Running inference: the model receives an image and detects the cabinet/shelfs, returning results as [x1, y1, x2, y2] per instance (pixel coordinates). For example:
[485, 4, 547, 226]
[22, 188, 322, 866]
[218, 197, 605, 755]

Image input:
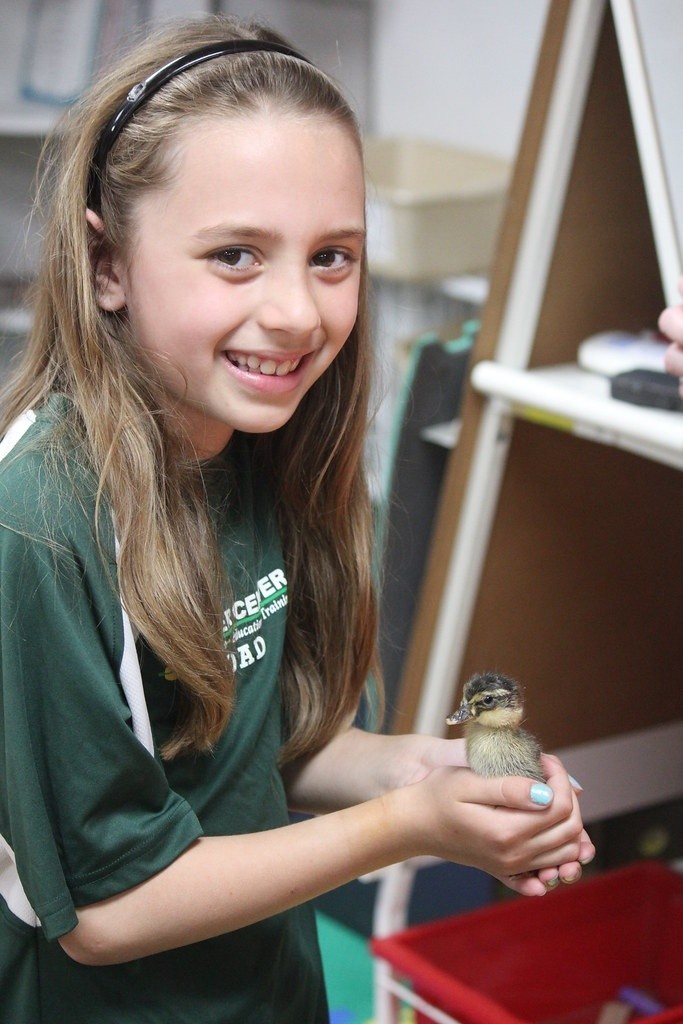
[0, 1, 375, 400]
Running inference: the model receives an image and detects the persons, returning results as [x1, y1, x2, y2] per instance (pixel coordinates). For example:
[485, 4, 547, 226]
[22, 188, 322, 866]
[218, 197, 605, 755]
[0, 14, 594, 1024]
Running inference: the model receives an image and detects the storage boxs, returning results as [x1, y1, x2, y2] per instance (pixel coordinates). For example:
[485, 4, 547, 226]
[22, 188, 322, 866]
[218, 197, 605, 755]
[370, 861, 683, 1024]
[351, 135, 516, 281]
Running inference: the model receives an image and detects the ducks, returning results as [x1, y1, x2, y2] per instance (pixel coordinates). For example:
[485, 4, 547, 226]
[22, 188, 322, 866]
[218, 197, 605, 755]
[444, 672, 547, 783]
